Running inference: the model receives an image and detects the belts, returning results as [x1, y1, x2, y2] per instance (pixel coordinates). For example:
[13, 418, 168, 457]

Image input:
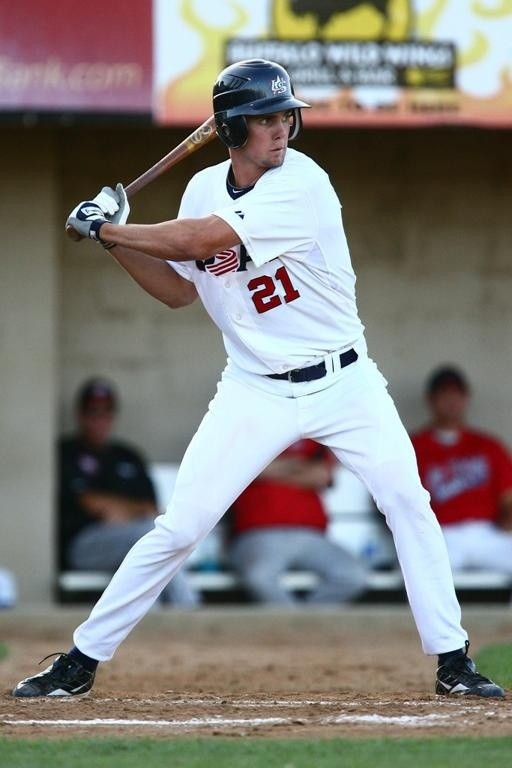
[264, 349, 358, 383]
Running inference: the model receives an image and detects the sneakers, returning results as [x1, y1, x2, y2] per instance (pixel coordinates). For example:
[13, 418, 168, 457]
[11, 653, 97, 700]
[435, 639, 504, 698]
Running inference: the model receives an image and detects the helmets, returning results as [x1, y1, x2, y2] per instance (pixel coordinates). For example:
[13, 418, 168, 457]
[212, 59, 314, 150]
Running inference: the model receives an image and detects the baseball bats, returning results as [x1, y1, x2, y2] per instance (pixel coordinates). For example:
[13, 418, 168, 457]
[66, 114, 218, 242]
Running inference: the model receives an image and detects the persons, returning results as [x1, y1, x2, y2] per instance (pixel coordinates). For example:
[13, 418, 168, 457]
[373, 356, 509, 606]
[41, 372, 202, 612]
[220, 436, 370, 605]
[1, 56, 510, 703]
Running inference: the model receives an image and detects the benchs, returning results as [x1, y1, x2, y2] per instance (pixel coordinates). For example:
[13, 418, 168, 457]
[58, 462, 512, 592]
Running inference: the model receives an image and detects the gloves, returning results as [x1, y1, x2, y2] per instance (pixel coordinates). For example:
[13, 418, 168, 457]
[64, 183, 131, 250]
